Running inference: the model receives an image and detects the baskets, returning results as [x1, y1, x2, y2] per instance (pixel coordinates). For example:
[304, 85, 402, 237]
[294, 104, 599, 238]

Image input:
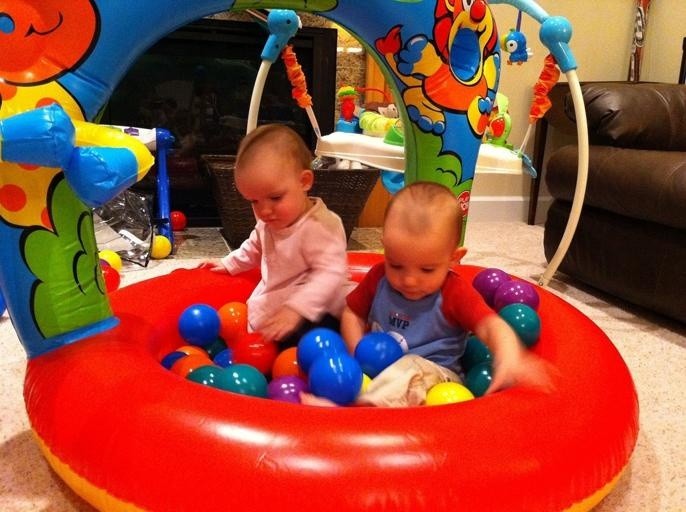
[200, 152, 382, 253]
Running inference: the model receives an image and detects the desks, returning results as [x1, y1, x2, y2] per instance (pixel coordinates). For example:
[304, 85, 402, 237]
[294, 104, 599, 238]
[100, 18, 338, 226]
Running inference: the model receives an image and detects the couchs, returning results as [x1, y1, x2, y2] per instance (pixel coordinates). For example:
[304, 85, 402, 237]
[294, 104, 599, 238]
[544, 83, 686, 326]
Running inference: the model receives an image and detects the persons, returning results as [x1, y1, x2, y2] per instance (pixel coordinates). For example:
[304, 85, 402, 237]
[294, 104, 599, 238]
[300, 181, 565, 409]
[196, 123, 360, 352]
